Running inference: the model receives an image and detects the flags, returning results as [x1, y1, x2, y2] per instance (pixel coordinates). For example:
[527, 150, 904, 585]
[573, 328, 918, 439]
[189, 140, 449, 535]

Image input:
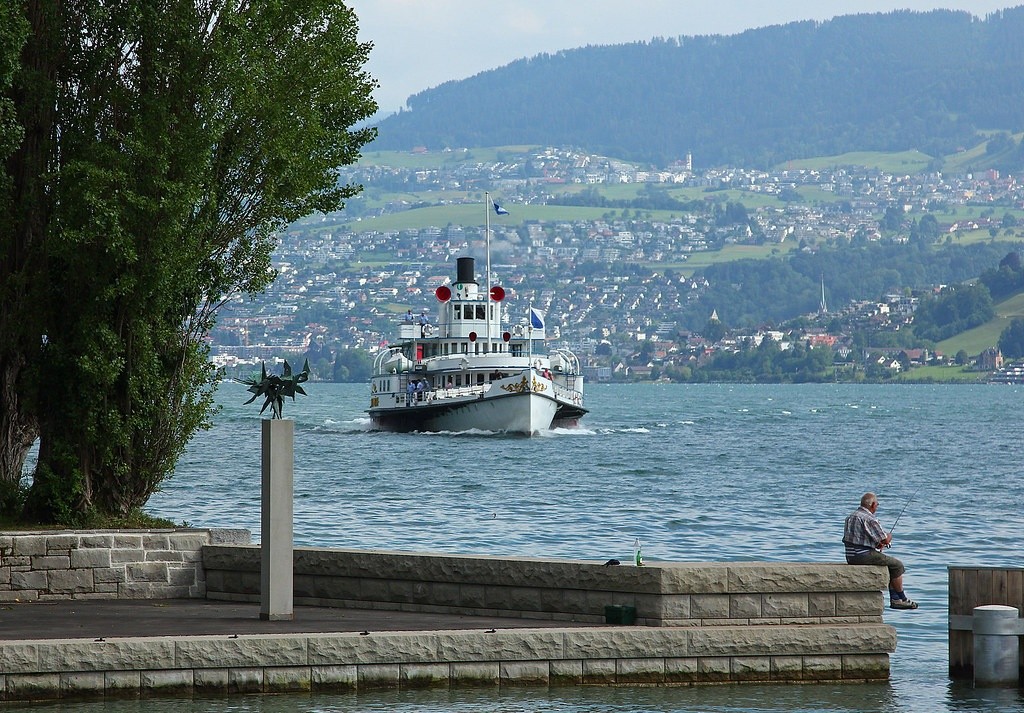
[489, 194, 510, 216]
[531, 306, 547, 329]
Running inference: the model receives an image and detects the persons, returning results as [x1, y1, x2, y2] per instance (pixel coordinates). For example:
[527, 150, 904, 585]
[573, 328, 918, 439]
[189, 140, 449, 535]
[542, 368, 553, 381]
[494, 369, 502, 379]
[446, 379, 453, 389]
[842, 492, 918, 610]
[419, 311, 430, 328]
[404, 309, 415, 325]
[407, 377, 432, 399]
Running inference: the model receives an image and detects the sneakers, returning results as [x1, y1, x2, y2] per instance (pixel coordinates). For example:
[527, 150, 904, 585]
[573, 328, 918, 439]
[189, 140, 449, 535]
[890, 597, 918, 609]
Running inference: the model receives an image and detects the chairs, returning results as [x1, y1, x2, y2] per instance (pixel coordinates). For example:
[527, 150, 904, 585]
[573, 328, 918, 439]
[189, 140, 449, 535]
[427, 383, 491, 400]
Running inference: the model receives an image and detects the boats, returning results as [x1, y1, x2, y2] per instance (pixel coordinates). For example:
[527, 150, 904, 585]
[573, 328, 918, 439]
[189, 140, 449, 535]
[383, 351, 413, 373]
[362, 191, 588, 435]
[550, 353, 574, 375]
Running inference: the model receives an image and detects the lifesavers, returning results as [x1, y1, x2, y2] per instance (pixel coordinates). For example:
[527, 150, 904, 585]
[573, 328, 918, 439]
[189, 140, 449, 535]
[512, 324, 524, 337]
[422, 323, 434, 336]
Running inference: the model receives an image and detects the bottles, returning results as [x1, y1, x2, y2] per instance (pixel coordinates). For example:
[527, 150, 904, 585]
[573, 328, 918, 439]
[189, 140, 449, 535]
[633, 537, 641, 565]
[636, 551, 642, 566]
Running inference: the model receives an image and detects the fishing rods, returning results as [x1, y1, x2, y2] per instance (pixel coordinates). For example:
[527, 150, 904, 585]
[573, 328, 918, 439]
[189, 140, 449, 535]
[883, 482, 927, 549]
